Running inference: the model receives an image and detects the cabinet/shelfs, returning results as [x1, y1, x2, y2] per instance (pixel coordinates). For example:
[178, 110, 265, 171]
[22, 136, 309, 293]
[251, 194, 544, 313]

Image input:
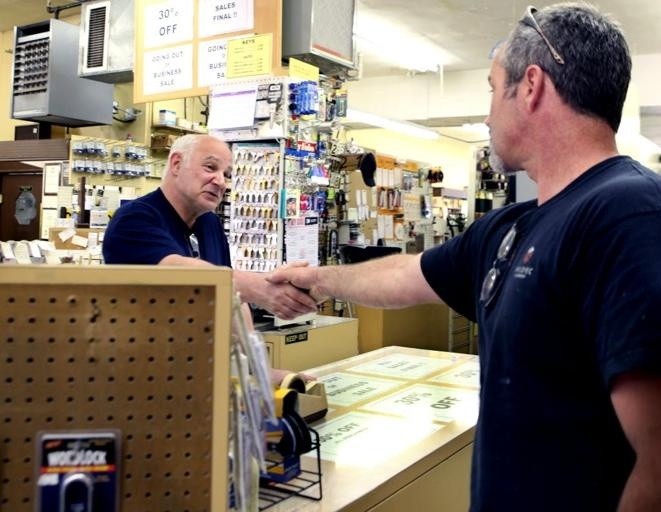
[342, 302, 470, 354]
[251, 316, 359, 375]
[469, 128, 660, 353]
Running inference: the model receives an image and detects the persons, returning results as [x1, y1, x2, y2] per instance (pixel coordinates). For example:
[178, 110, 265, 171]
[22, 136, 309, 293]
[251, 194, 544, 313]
[262, 1, 661, 511]
[100, 133, 321, 389]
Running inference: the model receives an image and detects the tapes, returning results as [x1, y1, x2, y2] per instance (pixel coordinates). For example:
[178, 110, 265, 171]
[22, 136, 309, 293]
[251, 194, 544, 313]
[279, 373, 306, 394]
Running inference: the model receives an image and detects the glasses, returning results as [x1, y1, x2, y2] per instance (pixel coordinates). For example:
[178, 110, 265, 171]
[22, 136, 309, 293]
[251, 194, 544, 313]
[517, 5, 566, 65]
[478, 211, 530, 311]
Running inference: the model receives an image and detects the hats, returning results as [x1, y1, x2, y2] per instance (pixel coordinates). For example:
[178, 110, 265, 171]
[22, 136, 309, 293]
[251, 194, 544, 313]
[358, 152, 378, 188]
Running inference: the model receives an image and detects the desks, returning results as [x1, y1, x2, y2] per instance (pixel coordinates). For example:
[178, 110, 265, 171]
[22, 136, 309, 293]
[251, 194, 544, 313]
[229, 344, 483, 512]
